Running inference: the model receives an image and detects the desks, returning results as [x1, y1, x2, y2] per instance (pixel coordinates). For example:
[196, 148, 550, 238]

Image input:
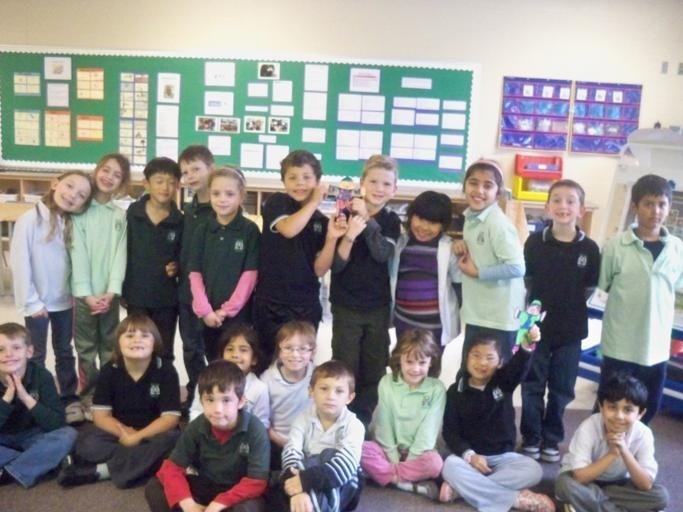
[0, 172, 598, 243]
[0, 203, 43, 297]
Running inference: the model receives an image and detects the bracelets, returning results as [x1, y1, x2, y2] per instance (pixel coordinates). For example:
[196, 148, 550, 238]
[344, 235, 354, 243]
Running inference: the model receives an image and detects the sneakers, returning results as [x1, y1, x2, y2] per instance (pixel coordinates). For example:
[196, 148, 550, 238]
[438, 481, 461, 503]
[542, 448, 560, 463]
[56, 465, 100, 488]
[412, 480, 439, 501]
[518, 489, 556, 512]
[65, 396, 92, 427]
[523, 446, 541, 460]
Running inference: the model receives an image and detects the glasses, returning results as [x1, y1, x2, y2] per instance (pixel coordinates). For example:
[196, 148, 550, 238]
[278, 346, 314, 353]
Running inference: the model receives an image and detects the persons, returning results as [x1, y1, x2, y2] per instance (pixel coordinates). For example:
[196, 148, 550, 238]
[328, 154, 401, 426]
[11, 169, 95, 423]
[0, 311, 668, 510]
[452, 160, 526, 383]
[519, 179, 602, 461]
[186, 166, 262, 364]
[65, 153, 129, 422]
[387, 190, 463, 379]
[120, 157, 184, 363]
[252, 150, 349, 378]
[177, 145, 217, 410]
[592, 175, 682, 425]
